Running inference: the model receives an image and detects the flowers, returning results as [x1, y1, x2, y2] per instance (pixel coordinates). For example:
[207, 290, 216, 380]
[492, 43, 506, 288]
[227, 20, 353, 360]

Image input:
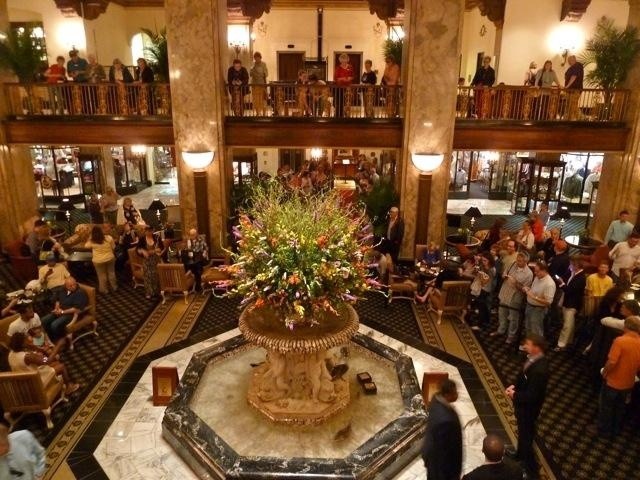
[208, 172, 394, 332]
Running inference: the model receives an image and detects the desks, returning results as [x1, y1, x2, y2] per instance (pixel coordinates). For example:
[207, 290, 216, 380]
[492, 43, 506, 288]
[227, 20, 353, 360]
[565, 235, 603, 255]
[33, 223, 66, 242]
[445, 234, 481, 261]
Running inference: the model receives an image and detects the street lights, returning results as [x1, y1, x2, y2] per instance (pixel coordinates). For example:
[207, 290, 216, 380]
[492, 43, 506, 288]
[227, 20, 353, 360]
[180, 150, 218, 258]
[411, 152, 446, 253]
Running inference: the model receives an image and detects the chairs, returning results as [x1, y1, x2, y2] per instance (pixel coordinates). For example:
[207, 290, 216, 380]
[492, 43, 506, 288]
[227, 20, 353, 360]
[387, 244, 472, 326]
[2, 229, 231, 305]
[1, 280, 99, 428]
[579, 245, 610, 269]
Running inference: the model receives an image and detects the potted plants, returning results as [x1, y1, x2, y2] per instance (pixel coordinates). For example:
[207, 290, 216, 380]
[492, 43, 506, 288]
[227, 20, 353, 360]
[580, 16, 640, 121]
[0, 23, 49, 115]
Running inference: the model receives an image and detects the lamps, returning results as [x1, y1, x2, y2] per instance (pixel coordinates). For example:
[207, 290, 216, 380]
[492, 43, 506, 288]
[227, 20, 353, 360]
[554, 209, 571, 239]
[411, 153, 444, 173]
[58, 199, 76, 235]
[181, 151, 214, 170]
[146, 198, 166, 225]
[464, 207, 483, 236]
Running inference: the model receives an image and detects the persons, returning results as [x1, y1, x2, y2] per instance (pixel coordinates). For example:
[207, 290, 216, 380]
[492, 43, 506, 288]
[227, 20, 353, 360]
[464, 199, 639, 358]
[348, 156, 475, 306]
[8, 333, 80, 395]
[504, 334, 549, 463]
[462, 434, 523, 480]
[332, 54, 355, 118]
[383, 55, 400, 120]
[26, 187, 209, 296]
[1, 252, 91, 367]
[43, 56, 66, 117]
[531, 60, 560, 123]
[563, 55, 583, 121]
[227, 59, 250, 117]
[420, 379, 463, 480]
[523, 61, 537, 86]
[457, 77, 465, 112]
[66, 51, 95, 114]
[132, 57, 154, 115]
[86, 51, 106, 116]
[227, 156, 331, 262]
[468, 55, 496, 116]
[249, 51, 268, 118]
[362, 60, 377, 117]
[0, 415, 45, 480]
[600, 315, 639, 435]
[108, 58, 135, 115]
[296, 72, 326, 119]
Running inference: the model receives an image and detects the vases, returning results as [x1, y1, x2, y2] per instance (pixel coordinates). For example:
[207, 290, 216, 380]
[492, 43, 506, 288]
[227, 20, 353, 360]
[238, 302, 359, 425]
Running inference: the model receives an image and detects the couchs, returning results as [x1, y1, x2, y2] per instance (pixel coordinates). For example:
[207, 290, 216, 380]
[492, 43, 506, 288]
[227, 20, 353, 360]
[64, 223, 147, 252]
